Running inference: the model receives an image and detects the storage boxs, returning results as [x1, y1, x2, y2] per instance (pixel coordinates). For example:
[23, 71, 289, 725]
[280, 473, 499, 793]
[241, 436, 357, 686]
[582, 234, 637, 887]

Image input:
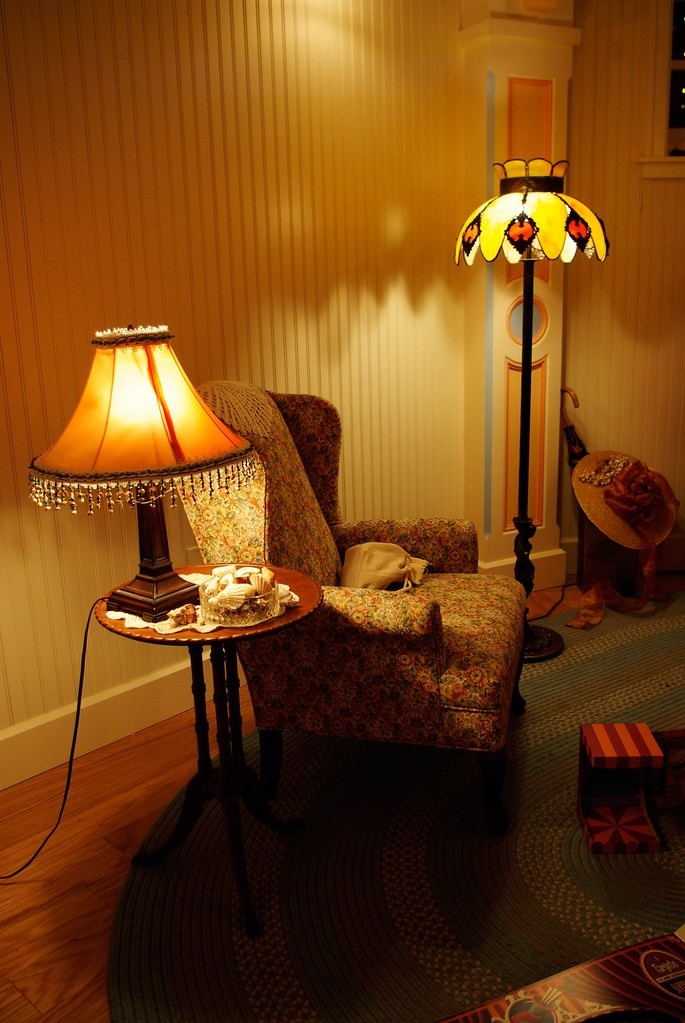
[577, 722, 663, 857]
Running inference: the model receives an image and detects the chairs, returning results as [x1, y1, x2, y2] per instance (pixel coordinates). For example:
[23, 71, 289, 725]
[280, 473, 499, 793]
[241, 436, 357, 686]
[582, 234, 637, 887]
[180, 379, 530, 840]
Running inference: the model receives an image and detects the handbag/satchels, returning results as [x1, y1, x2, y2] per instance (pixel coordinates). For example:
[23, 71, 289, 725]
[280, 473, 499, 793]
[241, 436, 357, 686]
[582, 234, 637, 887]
[339, 542, 429, 594]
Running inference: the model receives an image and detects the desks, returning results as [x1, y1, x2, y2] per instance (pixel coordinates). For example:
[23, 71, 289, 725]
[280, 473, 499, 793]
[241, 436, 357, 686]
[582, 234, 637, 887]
[95, 560, 324, 945]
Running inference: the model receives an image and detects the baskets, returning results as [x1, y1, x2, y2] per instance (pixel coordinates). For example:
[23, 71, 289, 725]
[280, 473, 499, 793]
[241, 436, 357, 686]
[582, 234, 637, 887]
[199, 577, 280, 628]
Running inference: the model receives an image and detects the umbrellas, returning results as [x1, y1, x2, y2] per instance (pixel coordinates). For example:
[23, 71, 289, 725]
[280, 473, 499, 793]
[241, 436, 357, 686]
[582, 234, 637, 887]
[560, 388, 590, 470]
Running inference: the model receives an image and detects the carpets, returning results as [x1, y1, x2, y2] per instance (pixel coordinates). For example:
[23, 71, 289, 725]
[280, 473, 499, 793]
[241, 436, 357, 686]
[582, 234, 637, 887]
[108, 591, 685, 1023]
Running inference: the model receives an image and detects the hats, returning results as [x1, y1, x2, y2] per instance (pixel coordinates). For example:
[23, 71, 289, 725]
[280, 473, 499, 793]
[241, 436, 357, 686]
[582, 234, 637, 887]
[572, 450, 678, 550]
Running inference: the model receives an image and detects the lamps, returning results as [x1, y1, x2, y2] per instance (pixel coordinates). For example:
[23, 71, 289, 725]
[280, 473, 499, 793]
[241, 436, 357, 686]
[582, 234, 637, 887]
[34, 324, 260, 623]
[453, 155, 610, 665]
